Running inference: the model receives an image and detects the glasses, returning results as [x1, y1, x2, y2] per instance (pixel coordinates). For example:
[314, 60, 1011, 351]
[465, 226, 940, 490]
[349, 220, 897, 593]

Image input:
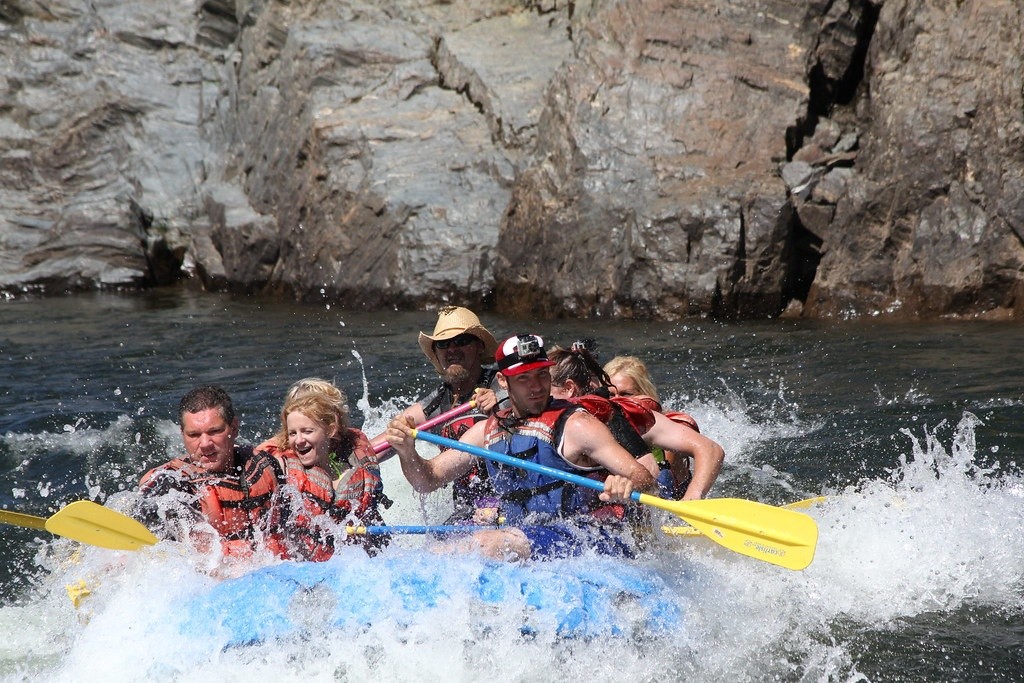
[435, 333, 480, 349]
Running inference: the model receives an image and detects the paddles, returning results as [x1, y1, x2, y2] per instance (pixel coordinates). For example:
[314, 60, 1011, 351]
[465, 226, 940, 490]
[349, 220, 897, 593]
[1, 503, 44, 544]
[373, 396, 476, 456]
[397, 426, 819, 569]
[345, 495, 841, 537]
[43, 498, 229, 579]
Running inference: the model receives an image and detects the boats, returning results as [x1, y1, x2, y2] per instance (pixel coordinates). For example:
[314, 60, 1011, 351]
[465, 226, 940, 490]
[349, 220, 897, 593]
[171, 555, 686, 658]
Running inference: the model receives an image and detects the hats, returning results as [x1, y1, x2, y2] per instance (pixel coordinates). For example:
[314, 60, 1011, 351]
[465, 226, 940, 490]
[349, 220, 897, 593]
[495, 332, 558, 377]
[417, 305, 500, 376]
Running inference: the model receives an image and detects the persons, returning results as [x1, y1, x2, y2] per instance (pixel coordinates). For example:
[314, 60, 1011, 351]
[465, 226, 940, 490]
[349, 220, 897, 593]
[370, 306, 511, 509]
[544, 342, 725, 518]
[384, 332, 653, 561]
[138, 383, 294, 560]
[251, 375, 392, 561]
